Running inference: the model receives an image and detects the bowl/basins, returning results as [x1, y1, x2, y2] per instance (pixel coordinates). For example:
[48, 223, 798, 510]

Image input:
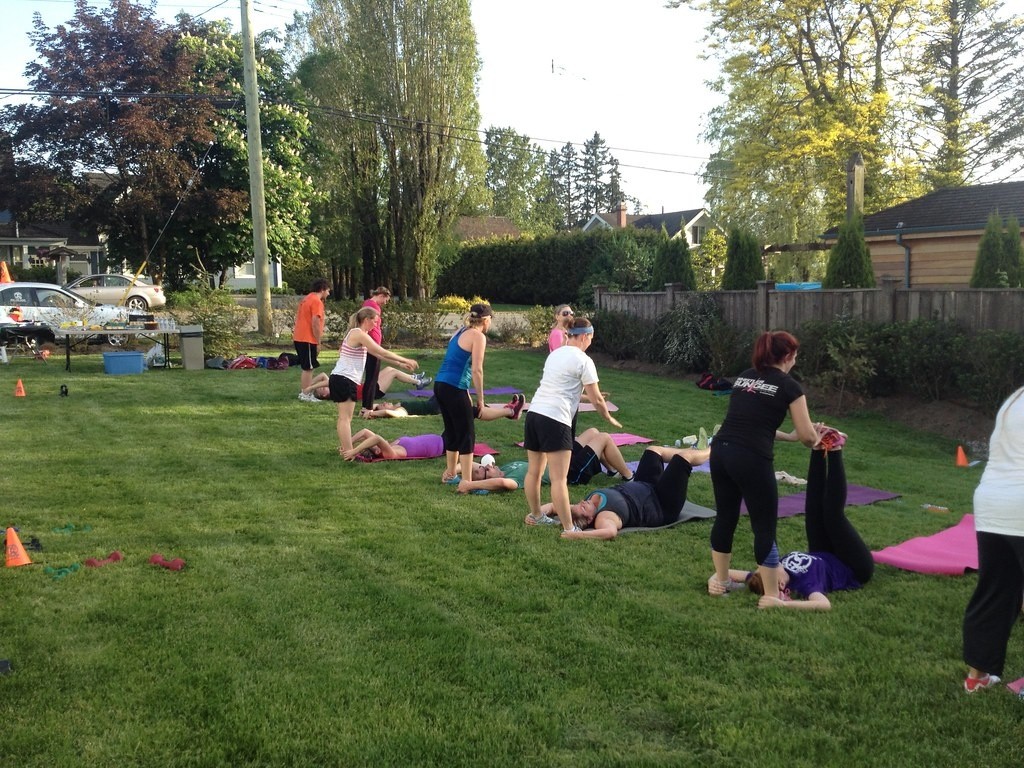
[143, 321, 158, 330]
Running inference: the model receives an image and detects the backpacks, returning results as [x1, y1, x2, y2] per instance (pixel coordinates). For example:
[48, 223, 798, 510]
[204, 352, 299, 370]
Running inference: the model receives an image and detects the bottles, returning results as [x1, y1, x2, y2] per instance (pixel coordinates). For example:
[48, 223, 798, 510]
[53, 308, 175, 330]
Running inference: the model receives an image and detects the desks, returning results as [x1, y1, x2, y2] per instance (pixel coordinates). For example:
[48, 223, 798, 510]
[55, 328, 180, 373]
[0, 323, 59, 365]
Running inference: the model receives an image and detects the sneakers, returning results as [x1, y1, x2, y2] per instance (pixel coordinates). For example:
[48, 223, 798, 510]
[298, 392, 322, 402]
[505, 394, 525, 420]
[713, 425, 722, 436]
[779, 591, 801, 603]
[411, 372, 432, 391]
[692, 426, 709, 451]
[530, 513, 561, 526]
[815, 425, 847, 458]
[719, 577, 744, 597]
[963, 675, 1000, 695]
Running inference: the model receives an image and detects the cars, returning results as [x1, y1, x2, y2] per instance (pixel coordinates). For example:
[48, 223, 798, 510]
[0, 282, 155, 347]
[63, 273, 167, 311]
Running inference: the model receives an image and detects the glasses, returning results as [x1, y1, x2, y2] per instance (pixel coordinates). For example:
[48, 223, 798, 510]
[478, 465, 488, 479]
[558, 310, 575, 317]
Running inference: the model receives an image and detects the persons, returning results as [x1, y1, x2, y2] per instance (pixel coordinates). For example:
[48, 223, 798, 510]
[361, 286, 390, 419]
[303, 365, 433, 401]
[340, 394, 525, 463]
[294, 280, 331, 402]
[709, 332, 831, 610]
[708, 426, 874, 609]
[526, 425, 721, 539]
[548, 305, 579, 442]
[92, 279, 99, 287]
[6, 262, 15, 282]
[442, 427, 635, 492]
[962, 388, 1024, 702]
[524, 318, 622, 532]
[329, 307, 419, 461]
[359, 395, 442, 420]
[434, 304, 494, 495]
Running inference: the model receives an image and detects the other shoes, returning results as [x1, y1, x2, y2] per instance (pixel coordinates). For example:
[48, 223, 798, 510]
[621, 470, 635, 483]
[446, 475, 462, 485]
[606, 469, 620, 479]
[467, 489, 490, 495]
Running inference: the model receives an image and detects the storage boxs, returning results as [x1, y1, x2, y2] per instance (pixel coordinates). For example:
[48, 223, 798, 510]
[102, 351, 144, 374]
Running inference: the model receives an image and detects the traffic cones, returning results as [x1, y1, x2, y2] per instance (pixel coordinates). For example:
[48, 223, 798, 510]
[14, 378, 26, 396]
[0, 261, 15, 283]
[957, 444, 969, 467]
[4, 526, 35, 568]
